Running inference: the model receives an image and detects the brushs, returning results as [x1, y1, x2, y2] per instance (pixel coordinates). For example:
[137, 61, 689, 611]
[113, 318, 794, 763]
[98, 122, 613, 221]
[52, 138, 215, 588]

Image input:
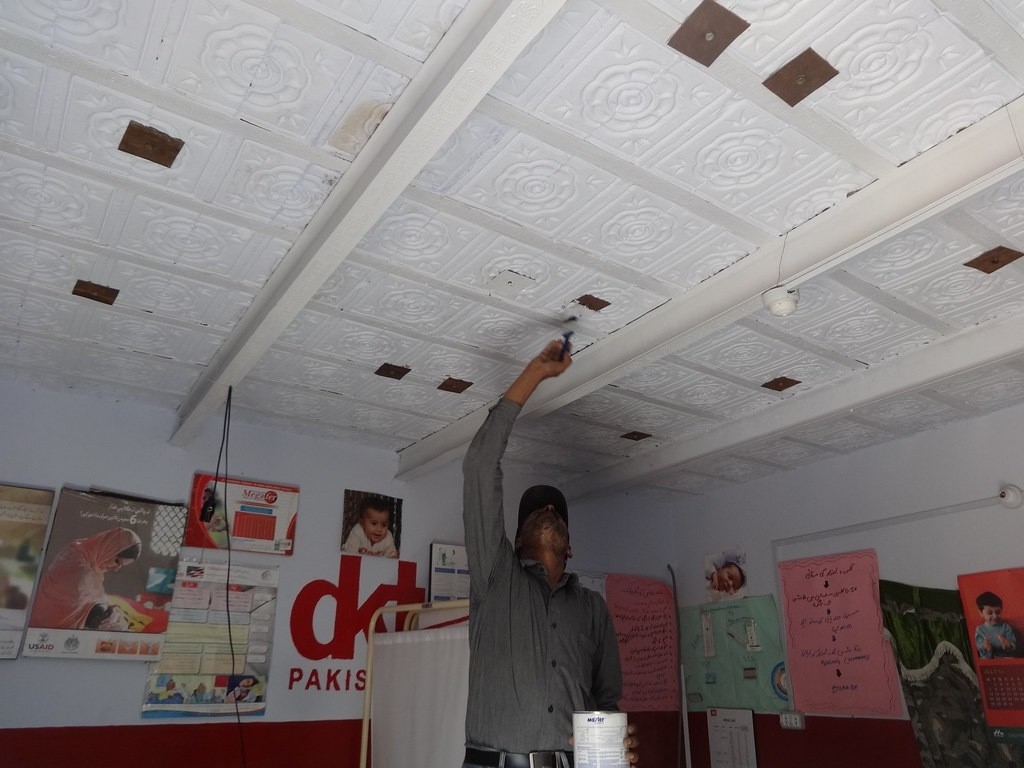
[559, 316, 578, 361]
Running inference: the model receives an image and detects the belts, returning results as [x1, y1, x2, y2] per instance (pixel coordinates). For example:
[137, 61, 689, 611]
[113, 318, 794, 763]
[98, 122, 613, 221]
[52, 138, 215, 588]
[464, 747, 574, 768]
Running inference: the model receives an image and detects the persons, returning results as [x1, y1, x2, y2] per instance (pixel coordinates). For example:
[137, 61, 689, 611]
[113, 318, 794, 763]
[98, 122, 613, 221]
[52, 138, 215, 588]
[30, 526, 142, 632]
[146, 675, 261, 704]
[707, 553, 747, 604]
[975, 591, 1019, 659]
[459, 339, 641, 768]
[199, 488, 226, 551]
[342, 496, 400, 559]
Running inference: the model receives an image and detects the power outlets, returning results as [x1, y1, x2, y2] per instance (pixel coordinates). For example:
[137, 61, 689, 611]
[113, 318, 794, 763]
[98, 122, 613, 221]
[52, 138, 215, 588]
[779, 712, 806, 729]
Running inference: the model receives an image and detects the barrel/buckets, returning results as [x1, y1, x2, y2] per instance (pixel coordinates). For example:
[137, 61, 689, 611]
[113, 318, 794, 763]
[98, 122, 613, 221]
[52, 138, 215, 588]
[572, 712, 628, 768]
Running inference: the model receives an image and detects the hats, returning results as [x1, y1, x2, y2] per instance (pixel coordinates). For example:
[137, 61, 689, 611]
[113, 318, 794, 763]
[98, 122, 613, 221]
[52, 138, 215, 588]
[518, 485, 569, 528]
